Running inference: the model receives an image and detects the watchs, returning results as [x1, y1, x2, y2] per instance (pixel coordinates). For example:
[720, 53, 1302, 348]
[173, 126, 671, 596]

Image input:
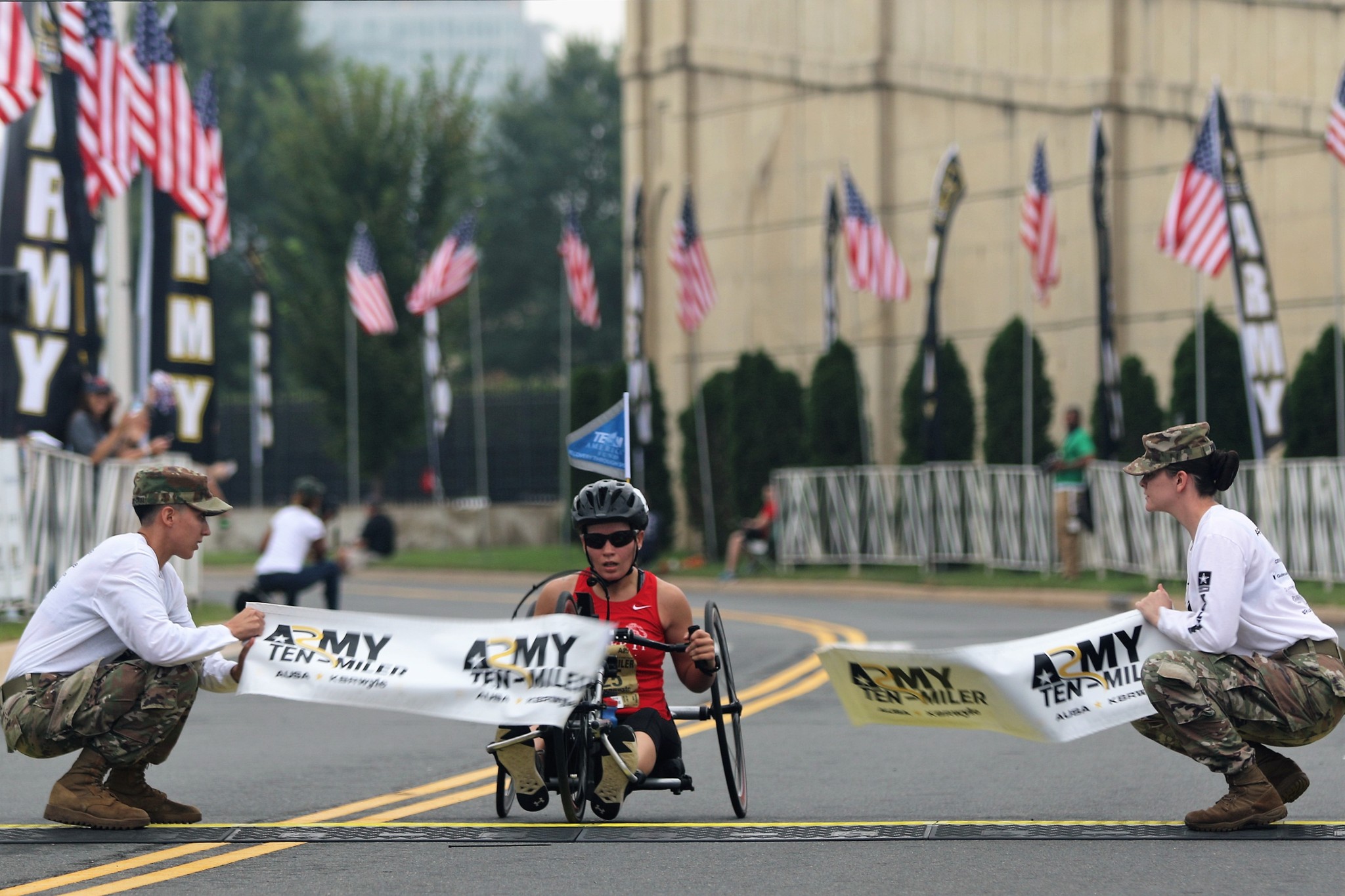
[694, 652, 721, 677]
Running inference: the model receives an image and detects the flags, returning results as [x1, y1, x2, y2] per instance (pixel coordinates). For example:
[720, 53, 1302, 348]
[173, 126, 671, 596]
[342, 219, 398, 337]
[1021, 140, 1060, 301]
[1158, 106, 1237, 284]
[842, 165, 913, 302]
[1210, 85, 1292, 436]
[560, 183, 601, 331]
[668, 184, 716, 335]
[1323, 61, 1344, 165]
[403, 199, 486, 316]
[0, 0, 277, 452]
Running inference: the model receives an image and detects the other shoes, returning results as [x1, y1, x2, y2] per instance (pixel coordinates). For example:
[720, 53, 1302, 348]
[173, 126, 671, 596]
[495, 725, 550, 812]
[591, 724, 639, 819]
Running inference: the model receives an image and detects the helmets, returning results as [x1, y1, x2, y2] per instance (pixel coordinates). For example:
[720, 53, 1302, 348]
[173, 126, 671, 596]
[570, 479, 649, 532]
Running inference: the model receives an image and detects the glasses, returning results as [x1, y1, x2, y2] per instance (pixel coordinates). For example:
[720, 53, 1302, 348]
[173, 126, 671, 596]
[584, 528, 643, 549]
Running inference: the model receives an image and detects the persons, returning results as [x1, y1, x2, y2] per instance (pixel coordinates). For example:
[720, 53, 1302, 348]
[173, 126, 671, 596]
[492, 477, 722, 821]
[1045, 405, 1095, 585]
[1, 466, 267, 829]
[60, 366, 397, 611]
[722, 484, 782, 585]
[1122, 422, 1345, 834]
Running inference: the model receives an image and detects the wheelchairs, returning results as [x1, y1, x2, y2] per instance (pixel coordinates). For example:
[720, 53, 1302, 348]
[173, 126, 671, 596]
[486, 569, 751, 821]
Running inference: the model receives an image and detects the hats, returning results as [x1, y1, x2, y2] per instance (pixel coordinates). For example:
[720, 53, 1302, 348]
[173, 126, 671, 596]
[1122, 421, 1216, 476]
[132, 464, 235, 515]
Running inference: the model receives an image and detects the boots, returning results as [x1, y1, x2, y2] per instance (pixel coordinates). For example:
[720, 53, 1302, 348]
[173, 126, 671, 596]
[1255, 745, 1310, 806]
[44, 744, 151, 829]
[1185, 765, 1288, 831]
[103, 767, 203, 824]
